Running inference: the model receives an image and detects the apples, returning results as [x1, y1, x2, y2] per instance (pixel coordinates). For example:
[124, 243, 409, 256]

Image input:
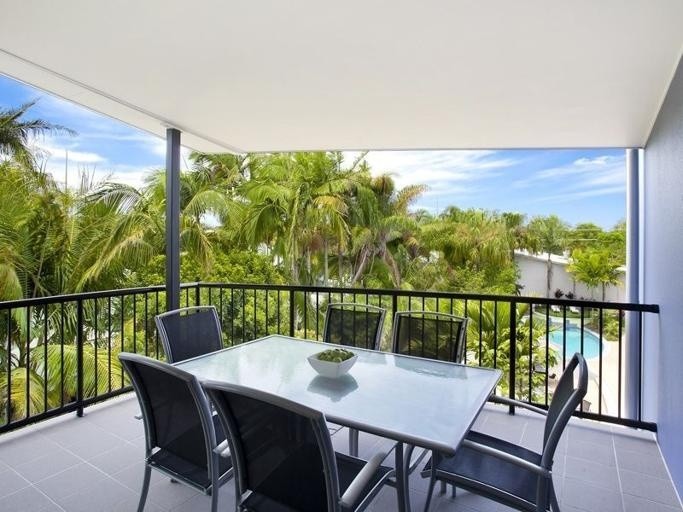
[318, 348, 354, 362]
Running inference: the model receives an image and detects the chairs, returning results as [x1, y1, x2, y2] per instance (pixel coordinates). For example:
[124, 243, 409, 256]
[323, 303, 386, 351]
[202, 378, 406, 512]
[352, 309, 469, 506]
[420, 351, 588, 512]
[118, 351, 234, 512]
[154, 305, 225, 362]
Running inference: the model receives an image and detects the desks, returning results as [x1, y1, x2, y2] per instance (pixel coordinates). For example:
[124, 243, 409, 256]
[169, 333, 504, 511]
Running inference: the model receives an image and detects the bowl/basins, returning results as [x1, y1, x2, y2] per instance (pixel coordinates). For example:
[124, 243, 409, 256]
[307, 375, 359, 402]
[307, 350, 358, 377]
